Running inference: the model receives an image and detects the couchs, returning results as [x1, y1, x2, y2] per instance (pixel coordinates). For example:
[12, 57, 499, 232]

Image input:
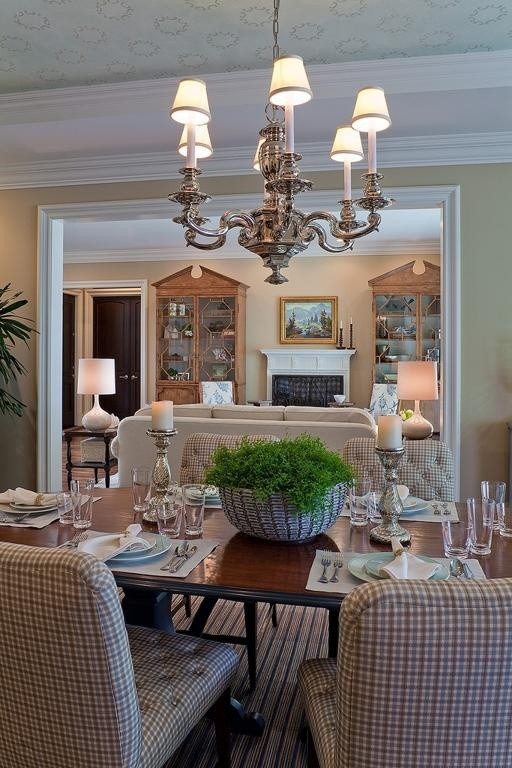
[115, 417, 375, 488]
[135, 408, 373, 423]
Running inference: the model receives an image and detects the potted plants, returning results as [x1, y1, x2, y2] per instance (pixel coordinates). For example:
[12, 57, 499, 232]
[200, 431, 360, 543]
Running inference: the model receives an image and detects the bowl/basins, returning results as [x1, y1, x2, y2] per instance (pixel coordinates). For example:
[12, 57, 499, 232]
[332, 394, 345, 402]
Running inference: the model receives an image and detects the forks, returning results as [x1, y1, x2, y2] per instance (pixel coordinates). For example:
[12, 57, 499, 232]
[0, 512, 30, 523]
[318, 549, 331, 582]
[331, 552, 343, 583]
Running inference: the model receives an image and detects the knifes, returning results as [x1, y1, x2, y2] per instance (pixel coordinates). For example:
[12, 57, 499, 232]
[463, 563, 472, 578]
[171, 546, 197, 573]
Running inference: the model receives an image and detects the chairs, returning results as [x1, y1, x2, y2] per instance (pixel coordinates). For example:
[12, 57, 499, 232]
[369, 384, 403, 423]
[297, 578, 512, 768]
[343, 439, 453, 502]
[181, 434, 278, 487]
[199, 381, 235, 405]
[0, 542, 239, 768]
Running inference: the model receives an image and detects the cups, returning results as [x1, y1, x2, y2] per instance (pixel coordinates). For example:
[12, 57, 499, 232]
[465, 497, 495, 556]
[57, 492, 78, 525]
[130, 467, 150, 512]
[441, 520, 470, 558]
[180, 484, 206, 537]
[479, 480, 505, 528]
[157, 505, 181, 539]
[370, 493, 382, 524]
[496, 502, 511, 537]
[348, 480, 370, 528]
[69, 480, 93, 529]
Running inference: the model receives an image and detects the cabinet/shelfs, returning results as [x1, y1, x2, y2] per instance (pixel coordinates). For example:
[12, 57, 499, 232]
[151, 266, 250, 404]
[369, 259, 441, 409]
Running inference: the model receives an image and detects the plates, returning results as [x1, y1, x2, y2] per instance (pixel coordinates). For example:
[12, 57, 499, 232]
[370, 499, 430, 514]
[77, 529, 173, 564]
[347, 552, 450, 584]
[3, 499, 63, 514]
[187, 486, 220, 502]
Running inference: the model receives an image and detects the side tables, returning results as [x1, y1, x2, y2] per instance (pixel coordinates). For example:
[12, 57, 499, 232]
[63, 425, 118, 489]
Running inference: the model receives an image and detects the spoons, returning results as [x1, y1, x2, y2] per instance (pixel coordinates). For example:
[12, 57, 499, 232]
[450, 559, 464, 579]
[160, 540, 189, 570]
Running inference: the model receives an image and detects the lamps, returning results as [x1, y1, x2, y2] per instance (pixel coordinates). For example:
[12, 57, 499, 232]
[397, 361, 438, 437]
[166, 2, 395, 286]
[77, 358, 116, 431]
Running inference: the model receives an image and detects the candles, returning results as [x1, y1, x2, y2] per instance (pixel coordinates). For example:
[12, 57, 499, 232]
[151, 401, 174, 432]
[378, 415, 402, 448]
[340, 320, 343, 328]
[351, 317, 352, 324]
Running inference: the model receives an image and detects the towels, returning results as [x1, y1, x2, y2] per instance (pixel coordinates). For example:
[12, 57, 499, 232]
[396, 484, 410, 502]
[379, 537, 441, 580]
[119, 524, 142, 546]
[0, 487, 66, 506]
[201, 484, 217, 495]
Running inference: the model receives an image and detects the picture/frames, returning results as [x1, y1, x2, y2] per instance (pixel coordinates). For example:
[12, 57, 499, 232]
[280, 297, 338, 346]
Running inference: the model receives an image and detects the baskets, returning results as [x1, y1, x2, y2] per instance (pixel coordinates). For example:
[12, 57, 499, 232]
[219, 484, 347, 544]
